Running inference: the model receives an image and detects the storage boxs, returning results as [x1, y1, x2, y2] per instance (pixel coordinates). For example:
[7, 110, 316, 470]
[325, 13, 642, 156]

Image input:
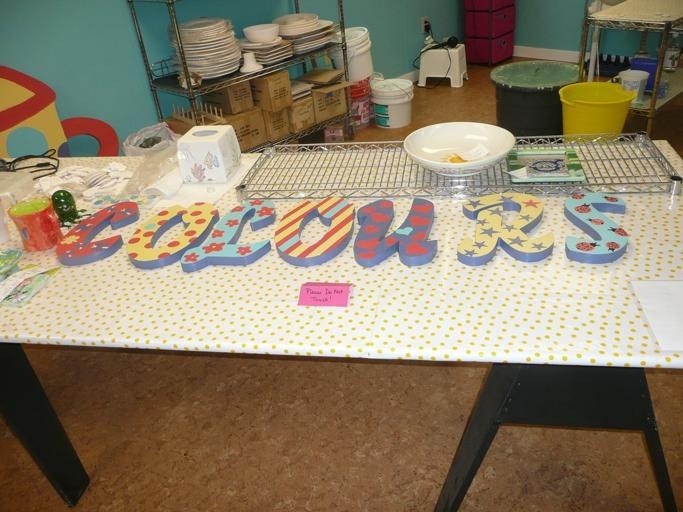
[164, 69, 352, 153]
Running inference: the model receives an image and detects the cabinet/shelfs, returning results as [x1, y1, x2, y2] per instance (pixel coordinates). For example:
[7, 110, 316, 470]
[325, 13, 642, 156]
[578, 0, 683, 139]
[127, 1, 352, 153]
[463, 1, 516, 67]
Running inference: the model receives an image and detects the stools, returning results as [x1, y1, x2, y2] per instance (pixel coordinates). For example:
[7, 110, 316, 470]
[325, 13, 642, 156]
[416, 43, 470, 88]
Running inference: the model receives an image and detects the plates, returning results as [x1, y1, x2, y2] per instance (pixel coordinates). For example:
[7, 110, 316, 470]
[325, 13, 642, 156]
[173, 17, 240, 81]
[241, 13, 335, 64]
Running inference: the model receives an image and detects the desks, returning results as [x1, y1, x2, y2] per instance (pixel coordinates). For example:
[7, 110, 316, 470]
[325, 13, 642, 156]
[0, 138, 682, 512]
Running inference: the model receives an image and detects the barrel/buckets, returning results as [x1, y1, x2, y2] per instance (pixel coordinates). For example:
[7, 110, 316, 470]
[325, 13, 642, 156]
[326, 27, 374, 84]
[344, 77, 373, 131]
[662, 47, 681, 71]
[620, 70, 649, 106]
[629, 56, 660, 94]
[559, 82, 638, 145]
[371, 78, 415, 128]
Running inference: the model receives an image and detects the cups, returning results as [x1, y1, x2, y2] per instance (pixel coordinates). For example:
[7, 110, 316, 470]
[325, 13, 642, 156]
[7, 198, 65, 252]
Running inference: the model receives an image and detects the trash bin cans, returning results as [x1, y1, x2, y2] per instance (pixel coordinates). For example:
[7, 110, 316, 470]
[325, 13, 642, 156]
[123, 126, 175, 153]
[489, 60, 580, 145]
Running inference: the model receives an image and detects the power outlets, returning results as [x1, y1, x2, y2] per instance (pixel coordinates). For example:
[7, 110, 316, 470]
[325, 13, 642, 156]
[421, 16, 430, 34]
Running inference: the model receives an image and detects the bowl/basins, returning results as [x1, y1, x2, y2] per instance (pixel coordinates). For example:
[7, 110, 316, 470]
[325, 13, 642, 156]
[403, 122, 516, 177]
